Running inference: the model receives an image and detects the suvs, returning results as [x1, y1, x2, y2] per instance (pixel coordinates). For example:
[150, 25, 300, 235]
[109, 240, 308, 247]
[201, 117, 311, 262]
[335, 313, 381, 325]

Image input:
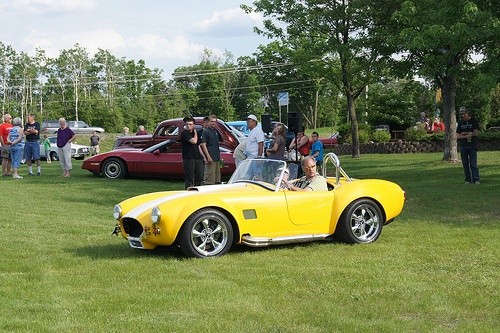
[112, 117, 240, 150]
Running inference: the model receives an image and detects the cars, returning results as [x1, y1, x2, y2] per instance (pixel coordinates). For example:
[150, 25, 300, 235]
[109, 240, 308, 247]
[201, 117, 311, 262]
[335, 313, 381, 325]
[39, 136, 90, 161]
[309, 132, 342, 150]
[224, 121, 271, 149]
[40, 121, 105, 133]
[269, 122, 297, 151]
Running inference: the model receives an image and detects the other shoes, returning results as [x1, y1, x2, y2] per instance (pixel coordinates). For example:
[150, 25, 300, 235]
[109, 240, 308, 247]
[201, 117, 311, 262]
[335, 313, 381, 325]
[37, 172, 42, 176]
[13, 176, 24, 179]
[28, 173, 33, 176]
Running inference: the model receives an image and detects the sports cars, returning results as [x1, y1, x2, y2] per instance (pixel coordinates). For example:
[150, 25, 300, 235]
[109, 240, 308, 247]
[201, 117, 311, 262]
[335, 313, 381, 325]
[82, 138, 236, 179]
[111, 152, 407, 259]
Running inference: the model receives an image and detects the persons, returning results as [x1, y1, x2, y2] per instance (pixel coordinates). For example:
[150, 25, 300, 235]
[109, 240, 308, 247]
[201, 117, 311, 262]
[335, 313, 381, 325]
[91, 131, 100, 156]
[181, 116, 208, 190]
[197, 116, 209, 146]
[233, 133, 270, 169]
[289, 126, 309, 158]
[255, 165, 290, 188]
[7, 117, 25, 179]
[44, 135, 51, 164]
[267, 124, 285, 160]
[246, 114, 265, 159]
[456, 106, 481, 184]
[136, 125, 148, 135]
[202, 114, 224, 184]
[282, 155, 328, 191]
[57, 118, 76, 177]
[122, 127, 130, 136]
[23, 113, 42, 176]
[311, 131, 323, 176]
[0, 114, 14, 177]
[414, 111, 445, 134]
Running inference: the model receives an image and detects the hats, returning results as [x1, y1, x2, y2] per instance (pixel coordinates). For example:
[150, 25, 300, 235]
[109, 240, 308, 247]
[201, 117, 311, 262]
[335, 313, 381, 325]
[247, 115, 257, 121]
[459, 107, 468, 114]
[183, 116, 195, 122]
[277, 165, 290, 174]
